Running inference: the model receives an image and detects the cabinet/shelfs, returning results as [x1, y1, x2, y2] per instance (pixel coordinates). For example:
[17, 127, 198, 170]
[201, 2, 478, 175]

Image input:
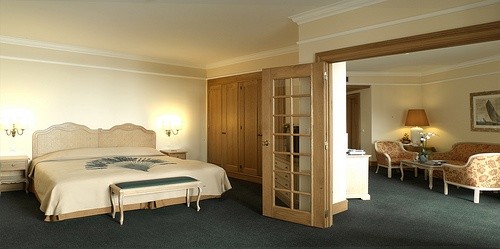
[207, 72, 285, 185]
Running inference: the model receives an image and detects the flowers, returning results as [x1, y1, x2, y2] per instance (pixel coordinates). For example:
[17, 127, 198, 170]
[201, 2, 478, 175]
[400, 133, 410, 141]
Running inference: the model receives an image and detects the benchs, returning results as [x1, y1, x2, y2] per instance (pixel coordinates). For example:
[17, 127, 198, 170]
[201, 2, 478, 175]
[109, 176, 204, 226]
[424, 141, 500, 203]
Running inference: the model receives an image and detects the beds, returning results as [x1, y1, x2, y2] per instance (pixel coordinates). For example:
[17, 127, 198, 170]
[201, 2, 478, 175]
[27, 122, 233, 222]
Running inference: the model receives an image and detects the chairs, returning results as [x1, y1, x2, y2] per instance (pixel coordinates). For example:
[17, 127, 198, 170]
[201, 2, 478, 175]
[374, 140, 420, 178]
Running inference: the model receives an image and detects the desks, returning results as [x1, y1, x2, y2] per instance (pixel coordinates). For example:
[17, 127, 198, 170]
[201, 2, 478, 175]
[346, 153, 372, 201]
[404, 144, 437, 153]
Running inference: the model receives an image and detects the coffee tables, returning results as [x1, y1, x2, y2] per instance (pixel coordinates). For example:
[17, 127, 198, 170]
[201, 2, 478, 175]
[397, 157, 465, 190]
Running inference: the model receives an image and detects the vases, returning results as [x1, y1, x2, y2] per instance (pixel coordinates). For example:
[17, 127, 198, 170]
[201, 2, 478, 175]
[402, 140, 411, 144]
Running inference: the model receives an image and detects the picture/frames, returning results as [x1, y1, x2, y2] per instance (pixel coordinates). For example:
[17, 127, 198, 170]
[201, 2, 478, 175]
[470, 90, 500, 132]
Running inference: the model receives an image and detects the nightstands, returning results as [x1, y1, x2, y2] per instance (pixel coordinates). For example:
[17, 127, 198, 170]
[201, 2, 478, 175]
[0, 156, 30, 194]
[160, 148, 187, 160]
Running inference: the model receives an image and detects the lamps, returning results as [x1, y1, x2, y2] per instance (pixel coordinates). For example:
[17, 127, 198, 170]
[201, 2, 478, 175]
[157, 116, 182, 137]
[405, 108, 430, 145]
[3, 123, 25, 137]
